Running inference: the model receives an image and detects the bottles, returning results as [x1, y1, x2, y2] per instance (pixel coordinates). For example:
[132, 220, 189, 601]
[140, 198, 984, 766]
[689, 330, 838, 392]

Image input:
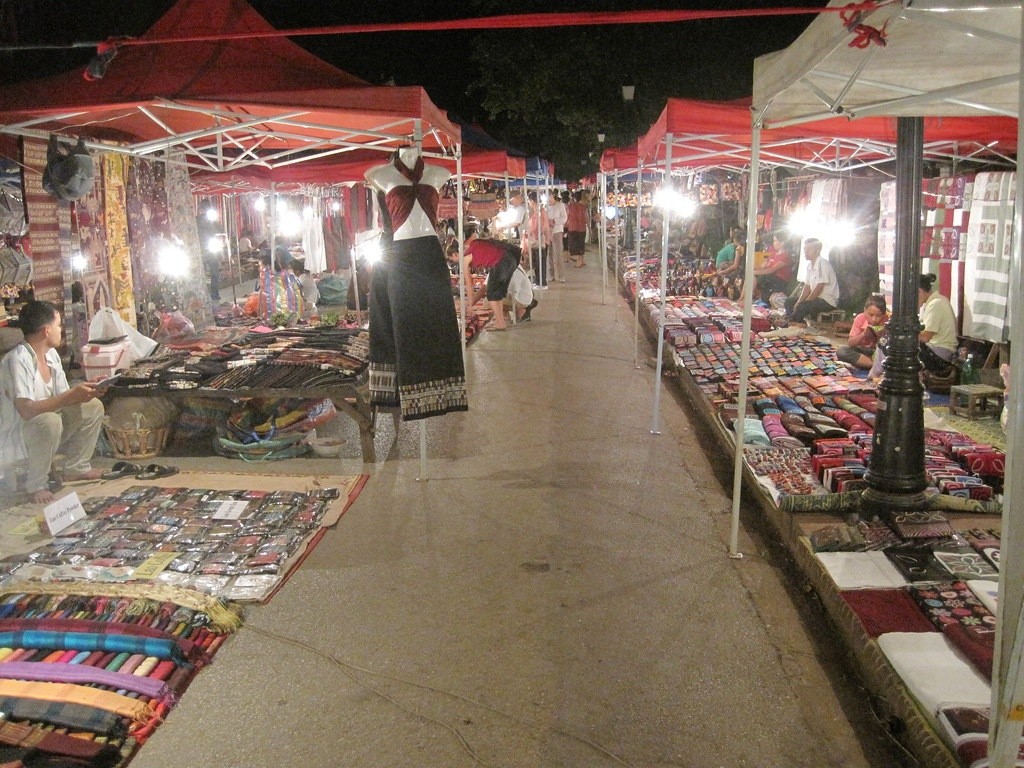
[853, 313, 857, 321]
[311, 304, 318, 314]
[482, 297, 488, 310]
[960, 354, 979, 407]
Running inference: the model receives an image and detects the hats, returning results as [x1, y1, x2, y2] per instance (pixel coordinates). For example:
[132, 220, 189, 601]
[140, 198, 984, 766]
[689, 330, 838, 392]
[509, 191, 520, 201]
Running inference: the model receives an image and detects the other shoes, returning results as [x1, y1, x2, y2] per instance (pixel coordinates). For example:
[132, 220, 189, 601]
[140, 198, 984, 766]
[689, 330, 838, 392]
[558, 277, 565, 282]
[533, 284, 548, 290]
[547, 277, 554, 281]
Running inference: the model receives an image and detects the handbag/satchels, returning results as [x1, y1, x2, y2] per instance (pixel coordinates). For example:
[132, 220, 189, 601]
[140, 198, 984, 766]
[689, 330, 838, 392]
[435, 176, 499, 221]
[472, 238, 522, 267]
[213, 398, 316, 465]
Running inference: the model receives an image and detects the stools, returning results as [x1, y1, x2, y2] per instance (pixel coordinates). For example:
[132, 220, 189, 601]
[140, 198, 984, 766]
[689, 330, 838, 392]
[816, 308, 845, 323]
[951, 385, 1005, 422]
[3, 456, 63, 492]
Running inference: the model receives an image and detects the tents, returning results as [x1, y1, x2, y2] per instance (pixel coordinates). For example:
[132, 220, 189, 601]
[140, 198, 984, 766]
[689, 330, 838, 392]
[191, 150, 532, 324]
[731, 0, 1024, 768]
[0, 1, 469, 476]
[601, 149, 751, 319]
[634, 94, 1018, 435]
[494, 159, 568, 284]
[581, 169, 690, 287]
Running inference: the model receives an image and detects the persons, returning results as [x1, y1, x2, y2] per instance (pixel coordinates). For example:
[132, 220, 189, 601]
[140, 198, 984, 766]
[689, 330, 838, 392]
[231, 232, 317, 314]
[197, 199, 225, 301]
[785, 237, 839, 327]
[0, 301, 113, 503]
[363, 148, 468, 421]
[837, 294, 888, 368]
[735, 233, 792, 304]
[437, 219, 538, 330]
[640, 209, 745, 294]
[511, 188, 601, 290]
[918, 273, 958, 372]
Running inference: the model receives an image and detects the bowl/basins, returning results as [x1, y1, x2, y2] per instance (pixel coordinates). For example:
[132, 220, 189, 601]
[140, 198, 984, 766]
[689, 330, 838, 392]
[308, 438, 348, 457]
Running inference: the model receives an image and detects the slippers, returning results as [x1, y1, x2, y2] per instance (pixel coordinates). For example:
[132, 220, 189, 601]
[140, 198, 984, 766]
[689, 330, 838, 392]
[485, 323, 506, 331]
[135, 464, 180, 480]
[509, 317, 531, 324]
[102, 462, 145, 480]
